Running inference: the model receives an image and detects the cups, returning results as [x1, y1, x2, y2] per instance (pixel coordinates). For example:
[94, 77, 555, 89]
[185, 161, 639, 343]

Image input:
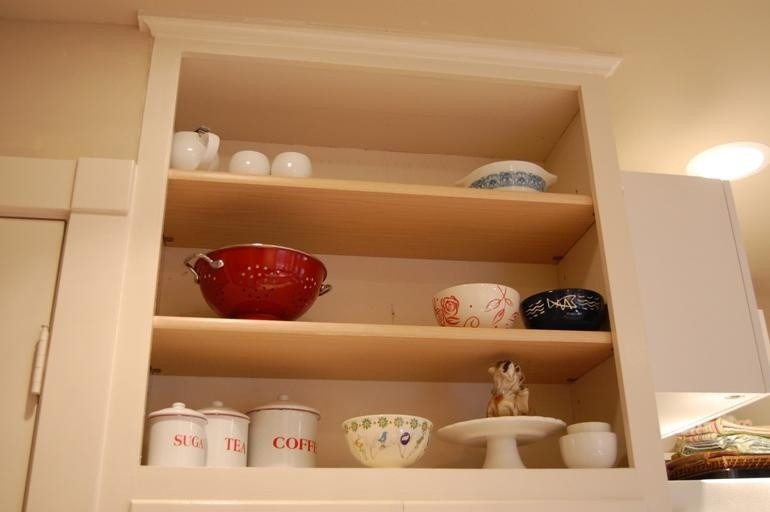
[245, 393, 322, 467]
[269, 152, 312, 177]
[169, 129, 220, 171]
[143, 402, 208, 467]
[227, 150, 271, 177]
[194, 400, 252, 467]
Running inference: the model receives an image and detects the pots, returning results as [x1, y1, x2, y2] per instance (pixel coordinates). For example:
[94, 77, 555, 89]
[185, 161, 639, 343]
[183, 242, 332, 322]
[455, 159, 559, 192]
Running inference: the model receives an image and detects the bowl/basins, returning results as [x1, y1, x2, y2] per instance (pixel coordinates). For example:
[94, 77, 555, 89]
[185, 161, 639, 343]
[558, 421, 618, 468]
[521, 287, 605, 330]
[430, 283, 520, 328]
[342, 413, 434, 468]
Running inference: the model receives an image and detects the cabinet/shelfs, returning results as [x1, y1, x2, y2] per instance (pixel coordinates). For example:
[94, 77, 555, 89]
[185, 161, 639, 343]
[107, 10, 637, 474]
[624, 172, 770, 439]
